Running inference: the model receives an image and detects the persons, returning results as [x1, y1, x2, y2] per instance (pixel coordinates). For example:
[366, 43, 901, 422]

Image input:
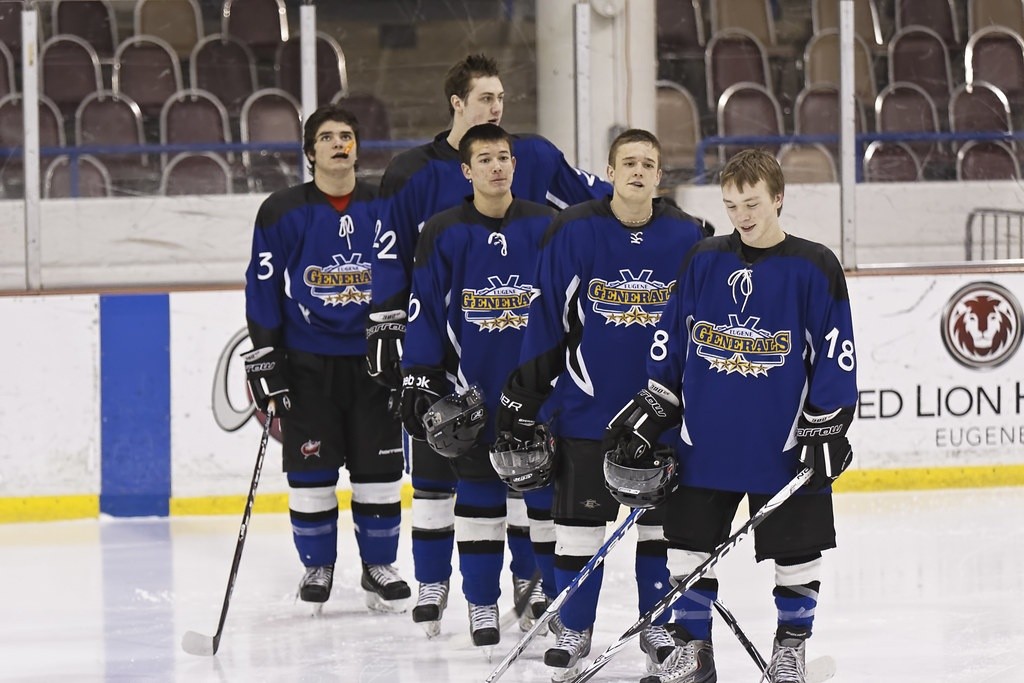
[605, 149, 858, 683]
[498, 129, 714, 683]
[244, 103, 411, 617]
[397, 122, 563, 662]
[364, 51, 714, 651]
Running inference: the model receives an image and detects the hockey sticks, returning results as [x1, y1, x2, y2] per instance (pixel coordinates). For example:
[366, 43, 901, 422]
[712, 595, 773, 683]
[484, 505, 652, 683]
[495, 569, 543, 635]
[181, 408, 276, 657]
[570, 465, 814, 683]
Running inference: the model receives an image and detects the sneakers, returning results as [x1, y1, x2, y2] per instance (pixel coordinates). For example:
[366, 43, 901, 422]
[295, 563, 334, 617]
[355, 530, 412, 614]
[639, 611, 676, 675]
[759, 638, 806, 683]
[465, 589, 500, 663]
[413, 579, 449, 637]
[510, 560, 550, 636]
[639, 619, 717, 683]
[544, 623, 593, 682]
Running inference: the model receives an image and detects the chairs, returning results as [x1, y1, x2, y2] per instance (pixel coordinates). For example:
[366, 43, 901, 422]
[656, 2, 1024, 179]
[1, 0, 391, 201]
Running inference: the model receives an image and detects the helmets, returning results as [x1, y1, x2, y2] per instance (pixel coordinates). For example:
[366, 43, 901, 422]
[492, 423, 555, 492]
[602, 441, 680, 510]
[422, 388, 489, 458]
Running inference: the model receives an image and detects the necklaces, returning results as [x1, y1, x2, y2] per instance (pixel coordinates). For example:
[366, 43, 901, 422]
[609, 198, 652, 224]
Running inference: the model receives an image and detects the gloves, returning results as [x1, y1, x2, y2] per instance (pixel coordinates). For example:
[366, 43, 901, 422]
[658, 196, 715, 237]
[239, 346, 293, 418]
[493, 367, 554, 451]
[602, 376, 680, 467]
[365, 302, 407, 391]
[793, 405, 853, 494]
[397, 366, 449, 441]
